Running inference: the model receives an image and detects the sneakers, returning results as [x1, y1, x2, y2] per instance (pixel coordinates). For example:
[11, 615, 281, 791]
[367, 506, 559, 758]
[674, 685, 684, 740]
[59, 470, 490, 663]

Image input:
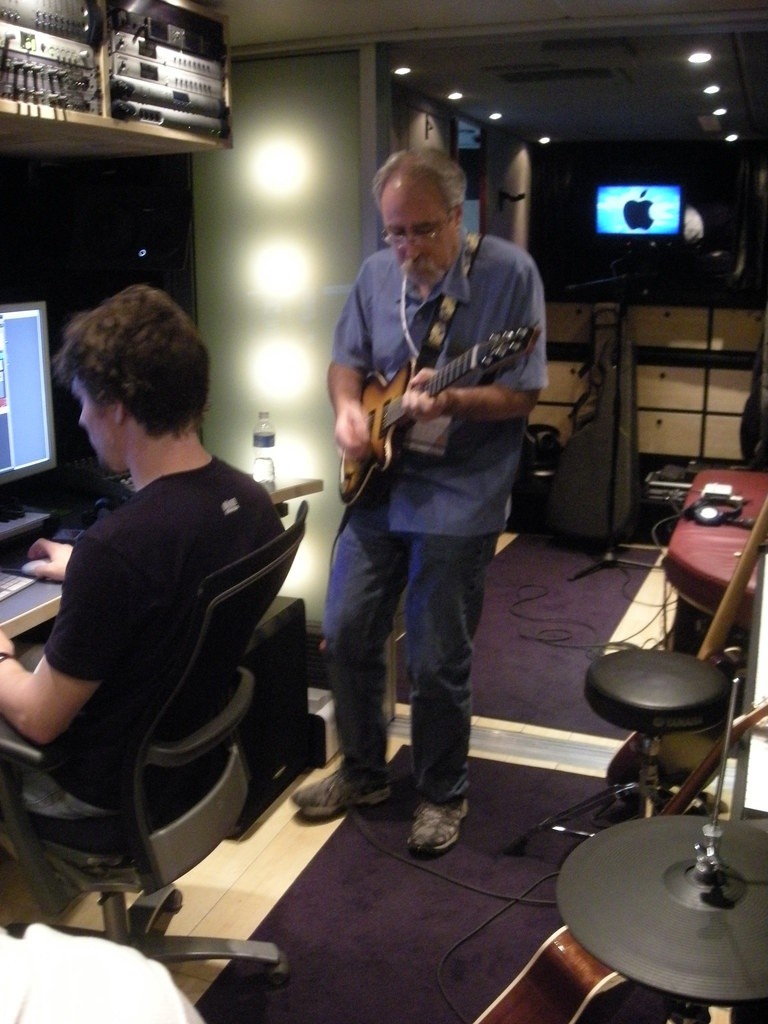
[292, 768, 392, 816]
[407, 797, 469, 852]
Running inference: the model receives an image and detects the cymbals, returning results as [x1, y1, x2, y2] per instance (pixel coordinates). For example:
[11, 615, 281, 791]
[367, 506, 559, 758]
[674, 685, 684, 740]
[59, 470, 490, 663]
[554, 814, 767, 1003]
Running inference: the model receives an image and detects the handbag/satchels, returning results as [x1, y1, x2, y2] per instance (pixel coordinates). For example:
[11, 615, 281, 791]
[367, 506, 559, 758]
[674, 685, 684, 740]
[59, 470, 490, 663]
[503, 423, 563, 532]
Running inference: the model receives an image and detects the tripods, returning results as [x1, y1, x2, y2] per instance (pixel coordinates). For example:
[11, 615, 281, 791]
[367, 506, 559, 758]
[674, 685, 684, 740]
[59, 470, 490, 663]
[565, 270, 661, 581]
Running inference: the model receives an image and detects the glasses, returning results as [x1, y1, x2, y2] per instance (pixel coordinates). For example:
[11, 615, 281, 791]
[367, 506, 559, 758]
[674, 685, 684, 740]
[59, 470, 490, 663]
[380, 209, 453, 250]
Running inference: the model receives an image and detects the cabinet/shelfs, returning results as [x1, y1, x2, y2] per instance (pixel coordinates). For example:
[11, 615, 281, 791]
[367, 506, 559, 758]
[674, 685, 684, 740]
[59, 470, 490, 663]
[0, 0, 236, 155]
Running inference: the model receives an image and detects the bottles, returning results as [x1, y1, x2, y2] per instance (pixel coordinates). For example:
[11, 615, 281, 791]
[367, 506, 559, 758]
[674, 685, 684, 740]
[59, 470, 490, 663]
[252, 411, 276, 483]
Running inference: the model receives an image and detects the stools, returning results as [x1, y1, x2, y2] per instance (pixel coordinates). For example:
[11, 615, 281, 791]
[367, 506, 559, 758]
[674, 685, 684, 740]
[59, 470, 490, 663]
[511, 649, 738, 861]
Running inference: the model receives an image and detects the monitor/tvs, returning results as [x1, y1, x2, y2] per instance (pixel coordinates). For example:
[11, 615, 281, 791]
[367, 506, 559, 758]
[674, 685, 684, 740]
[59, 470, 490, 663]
[596, 186, 682, 236]
[0, 301, 58, 521]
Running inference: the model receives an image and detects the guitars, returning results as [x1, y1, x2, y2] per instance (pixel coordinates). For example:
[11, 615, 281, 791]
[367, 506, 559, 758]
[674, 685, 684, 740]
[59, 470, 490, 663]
[472, 697, 768, 1024]
[338, 325, 542, 511]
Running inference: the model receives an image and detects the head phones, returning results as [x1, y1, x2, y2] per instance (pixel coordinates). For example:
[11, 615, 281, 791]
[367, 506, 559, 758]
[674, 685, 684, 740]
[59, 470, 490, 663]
[683, 497, 743, 527]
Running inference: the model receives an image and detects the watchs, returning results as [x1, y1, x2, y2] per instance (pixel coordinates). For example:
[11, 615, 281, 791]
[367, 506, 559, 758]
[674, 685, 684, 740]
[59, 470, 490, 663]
[0, 652, 18, 664]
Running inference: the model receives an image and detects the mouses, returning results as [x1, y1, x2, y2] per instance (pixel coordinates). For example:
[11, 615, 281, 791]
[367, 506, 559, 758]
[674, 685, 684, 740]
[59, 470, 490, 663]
[22, 558, 51, 577]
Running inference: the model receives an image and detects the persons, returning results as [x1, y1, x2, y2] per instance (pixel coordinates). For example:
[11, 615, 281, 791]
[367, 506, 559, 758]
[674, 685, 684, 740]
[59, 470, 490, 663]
[0, 283, 286, 866]
[315, 147, 545, 858]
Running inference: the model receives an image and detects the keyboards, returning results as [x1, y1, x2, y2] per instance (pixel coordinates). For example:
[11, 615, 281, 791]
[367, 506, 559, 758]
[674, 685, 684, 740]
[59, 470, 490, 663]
[0, 572, 35, 603]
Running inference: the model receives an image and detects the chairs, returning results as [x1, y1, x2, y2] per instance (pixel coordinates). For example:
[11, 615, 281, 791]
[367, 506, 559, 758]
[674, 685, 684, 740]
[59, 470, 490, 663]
[0, 496, 313, 988]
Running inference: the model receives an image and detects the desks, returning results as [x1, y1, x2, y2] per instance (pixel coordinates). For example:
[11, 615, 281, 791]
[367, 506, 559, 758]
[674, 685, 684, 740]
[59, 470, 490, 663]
[0, 476, 325, 639]
[661, 469, 767, 674]
